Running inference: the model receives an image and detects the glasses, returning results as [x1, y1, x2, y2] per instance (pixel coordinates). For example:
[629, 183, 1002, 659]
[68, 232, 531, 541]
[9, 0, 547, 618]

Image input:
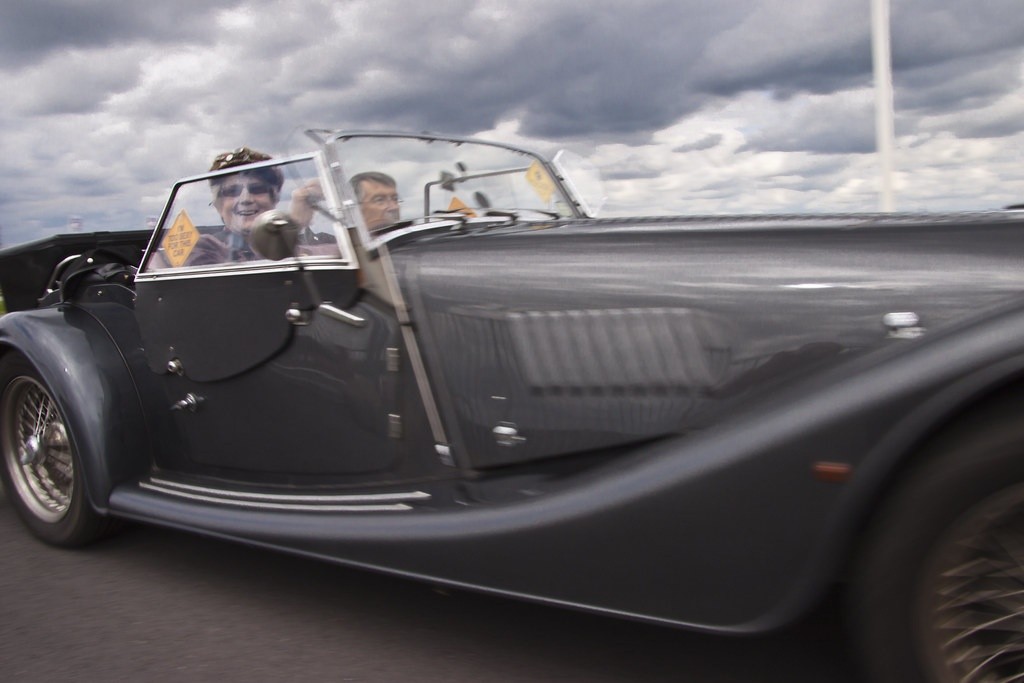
[218, 183, 274, 197]
[361, 193, 404, 209]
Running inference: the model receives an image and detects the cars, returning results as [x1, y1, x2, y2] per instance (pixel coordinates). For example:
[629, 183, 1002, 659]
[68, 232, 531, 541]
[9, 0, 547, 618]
[0, 124, 1021, 683]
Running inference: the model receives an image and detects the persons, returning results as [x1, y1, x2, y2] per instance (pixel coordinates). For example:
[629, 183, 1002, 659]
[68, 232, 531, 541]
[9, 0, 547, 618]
[347, 172, 400, 232]
[143, 147, 346, 270]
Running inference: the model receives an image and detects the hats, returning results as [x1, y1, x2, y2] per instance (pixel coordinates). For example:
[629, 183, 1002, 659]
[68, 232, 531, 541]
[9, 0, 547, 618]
[209, 147, 283, 191]
